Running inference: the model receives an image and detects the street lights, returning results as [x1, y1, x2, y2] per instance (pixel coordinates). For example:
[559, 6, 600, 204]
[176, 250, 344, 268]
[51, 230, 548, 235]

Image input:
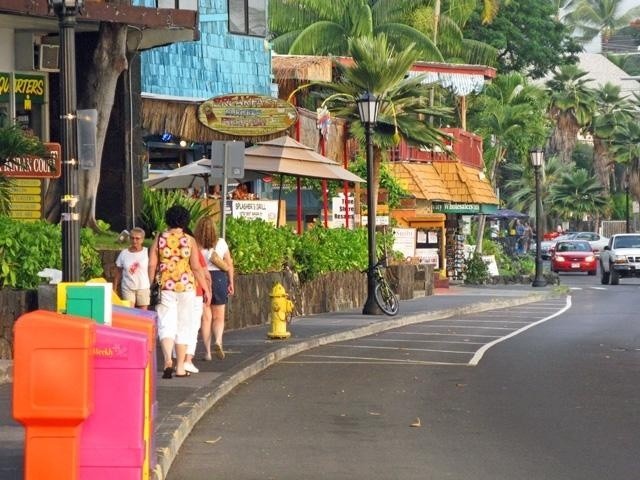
[48, 0, 84, 283]
[355, 81, 386, 315]
[528, 146, 546, 287]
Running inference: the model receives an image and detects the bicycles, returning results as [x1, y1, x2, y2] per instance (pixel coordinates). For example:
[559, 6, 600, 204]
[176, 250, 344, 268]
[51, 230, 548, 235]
[361, 256, 399, 316]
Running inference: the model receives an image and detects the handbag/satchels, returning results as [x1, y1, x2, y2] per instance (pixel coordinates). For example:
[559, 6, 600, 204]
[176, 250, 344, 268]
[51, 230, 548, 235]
[150, 281, 162, 306]
[210, 252, 229, 273]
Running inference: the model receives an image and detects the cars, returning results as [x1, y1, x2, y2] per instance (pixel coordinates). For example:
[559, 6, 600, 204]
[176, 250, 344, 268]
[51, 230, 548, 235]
[531, 231, 640, 284]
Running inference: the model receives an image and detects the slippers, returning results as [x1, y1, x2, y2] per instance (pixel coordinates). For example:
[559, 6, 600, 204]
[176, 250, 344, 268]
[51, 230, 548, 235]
[162, 366, 191, 379]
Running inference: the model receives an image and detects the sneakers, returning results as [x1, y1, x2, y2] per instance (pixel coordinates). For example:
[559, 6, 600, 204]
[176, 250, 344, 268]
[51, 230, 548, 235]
[204, 342, 225, 361]
[183, 362, 199, 374]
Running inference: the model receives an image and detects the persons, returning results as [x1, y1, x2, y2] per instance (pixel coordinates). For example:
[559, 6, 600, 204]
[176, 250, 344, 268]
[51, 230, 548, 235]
[112, 227, 151, 310]
[511, 218, 534, 255]
[488, 219, 499, 242]
[208, 184, 222, 197]
[148, 204, 234, 378]
[233, 184, 258, 201]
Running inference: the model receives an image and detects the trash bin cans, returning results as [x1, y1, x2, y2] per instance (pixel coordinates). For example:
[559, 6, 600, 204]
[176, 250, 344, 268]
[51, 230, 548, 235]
[541, 253, 551, 274]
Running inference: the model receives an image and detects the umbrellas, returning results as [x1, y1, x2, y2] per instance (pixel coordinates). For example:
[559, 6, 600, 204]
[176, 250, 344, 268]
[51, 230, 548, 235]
[144, 155, 272, 198]
[198, 129, 367, 228]
[470, 206, 529, 231]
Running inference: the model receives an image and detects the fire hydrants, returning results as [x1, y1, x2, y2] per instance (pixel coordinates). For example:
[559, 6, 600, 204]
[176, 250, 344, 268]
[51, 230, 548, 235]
[267, 282, 294, 339]
